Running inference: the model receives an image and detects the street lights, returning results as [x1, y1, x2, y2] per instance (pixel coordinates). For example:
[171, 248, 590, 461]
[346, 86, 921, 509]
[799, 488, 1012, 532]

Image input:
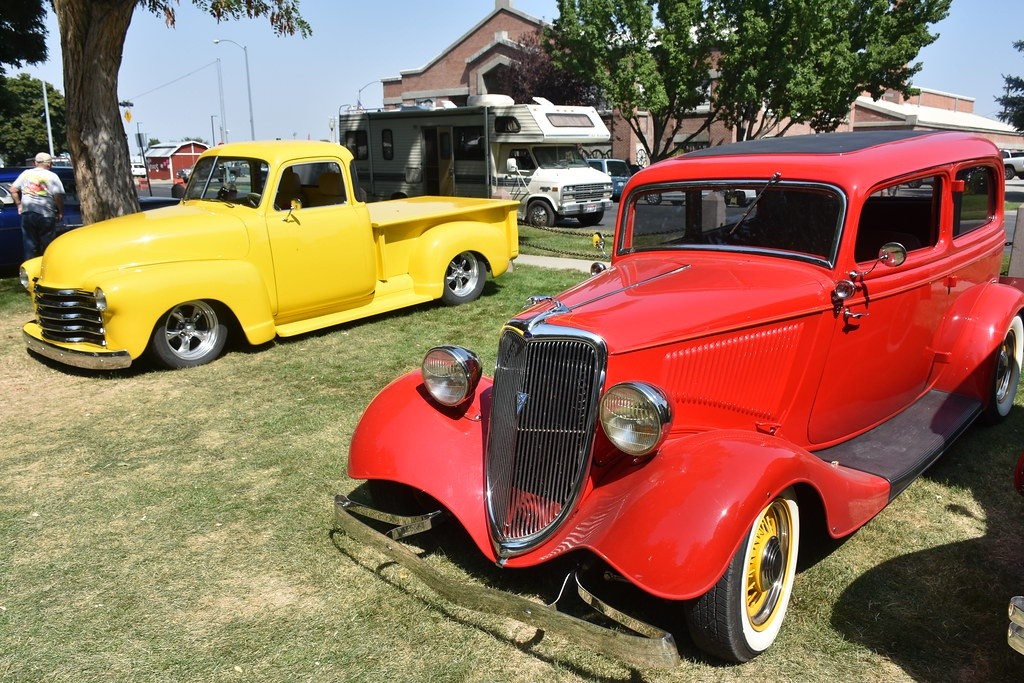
[211, 115, 218, 149]
[212, 38, 255, 142]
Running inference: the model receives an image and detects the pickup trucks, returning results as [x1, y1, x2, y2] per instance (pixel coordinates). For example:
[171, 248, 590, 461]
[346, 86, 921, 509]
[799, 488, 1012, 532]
[17, 138, 522, 379]
[998, 149, 1024, 181]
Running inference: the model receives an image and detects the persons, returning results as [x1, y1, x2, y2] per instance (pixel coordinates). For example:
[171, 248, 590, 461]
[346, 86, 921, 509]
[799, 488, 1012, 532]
[9, 152, 66, 262]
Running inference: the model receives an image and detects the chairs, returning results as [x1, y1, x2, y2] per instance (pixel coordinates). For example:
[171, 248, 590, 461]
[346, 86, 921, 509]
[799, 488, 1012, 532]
[274, 167, 302, 209]
[309, 172, 346, 207]
[859, 230, 921, 266]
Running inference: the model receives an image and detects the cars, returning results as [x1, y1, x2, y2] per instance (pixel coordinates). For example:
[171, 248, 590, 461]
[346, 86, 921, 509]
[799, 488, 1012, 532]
[0, 156, 184, 277]
[583, 158, 631, 202]
[344, 128, 1024, 664]
[627, 163, 686, 206]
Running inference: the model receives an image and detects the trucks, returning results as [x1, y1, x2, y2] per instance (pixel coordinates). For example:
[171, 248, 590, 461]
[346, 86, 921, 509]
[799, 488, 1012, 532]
[339, 94, 613, 229]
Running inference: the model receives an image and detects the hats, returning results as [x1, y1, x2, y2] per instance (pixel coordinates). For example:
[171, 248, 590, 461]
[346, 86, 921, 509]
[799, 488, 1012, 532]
[35, 152, 54, 165]
